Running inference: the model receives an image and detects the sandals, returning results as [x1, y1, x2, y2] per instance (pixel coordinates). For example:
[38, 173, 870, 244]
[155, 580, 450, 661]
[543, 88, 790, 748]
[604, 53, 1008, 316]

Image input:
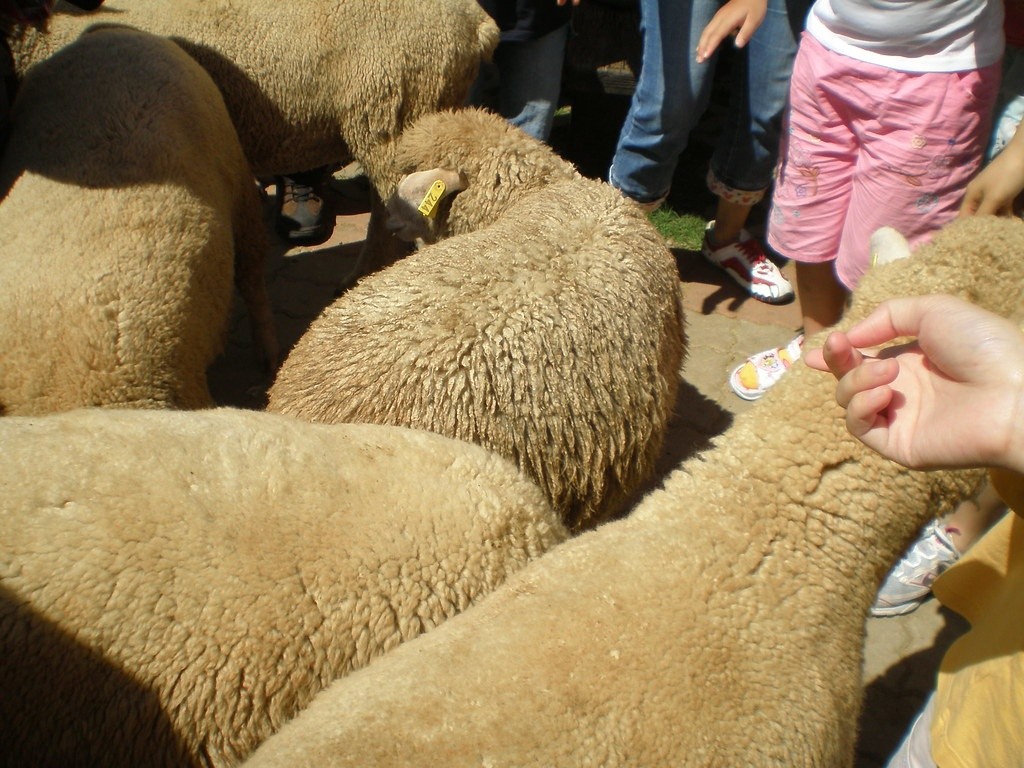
[730, 334, 808, 402]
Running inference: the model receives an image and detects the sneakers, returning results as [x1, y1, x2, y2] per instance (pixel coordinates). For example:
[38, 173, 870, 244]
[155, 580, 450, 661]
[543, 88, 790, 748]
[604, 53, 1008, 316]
[866, 520, 961, 617]
[276, 168, 339, 244]
[702, 220, 795, 305]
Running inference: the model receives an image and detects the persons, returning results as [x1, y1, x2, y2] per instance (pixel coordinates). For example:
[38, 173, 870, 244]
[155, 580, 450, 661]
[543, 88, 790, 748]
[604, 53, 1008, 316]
[269, 0, 1024, 309]
[694, 0, 1008, 399]
[863, 98, 1024, 621]
[819, 289, 1023, 768]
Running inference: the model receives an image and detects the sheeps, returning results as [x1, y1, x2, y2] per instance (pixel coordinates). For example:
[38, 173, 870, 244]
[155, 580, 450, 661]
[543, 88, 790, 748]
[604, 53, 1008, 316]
[0, 216, 1024, 768]
[0, 0, 686, 533]
